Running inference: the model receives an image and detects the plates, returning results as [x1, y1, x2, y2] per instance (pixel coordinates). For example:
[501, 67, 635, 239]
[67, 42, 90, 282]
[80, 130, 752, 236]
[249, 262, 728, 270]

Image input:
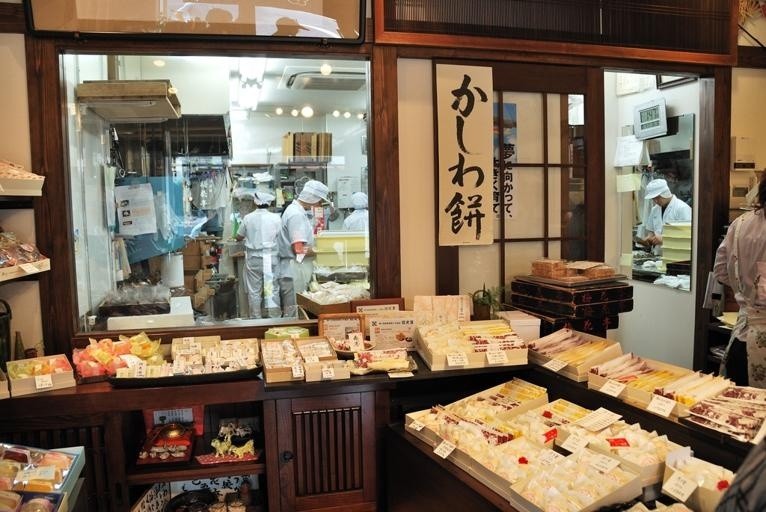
[335, 339, 376, 357]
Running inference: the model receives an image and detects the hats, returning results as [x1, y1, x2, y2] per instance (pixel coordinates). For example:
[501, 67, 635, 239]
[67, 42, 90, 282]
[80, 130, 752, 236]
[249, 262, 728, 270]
[644, 179, 672, 199]
[254, 191, 275, 206]
[351, 192, 368, 209]
[298, 181, 331, 204]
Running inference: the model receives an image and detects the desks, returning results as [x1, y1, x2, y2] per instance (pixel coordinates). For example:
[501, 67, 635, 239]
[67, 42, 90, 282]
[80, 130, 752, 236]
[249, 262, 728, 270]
[92, 296, 212, 331]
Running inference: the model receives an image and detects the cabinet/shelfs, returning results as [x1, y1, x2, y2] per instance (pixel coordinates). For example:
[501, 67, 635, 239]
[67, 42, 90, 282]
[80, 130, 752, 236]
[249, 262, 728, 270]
[0, 376, 394, 511]
[389, 361, 765, 509]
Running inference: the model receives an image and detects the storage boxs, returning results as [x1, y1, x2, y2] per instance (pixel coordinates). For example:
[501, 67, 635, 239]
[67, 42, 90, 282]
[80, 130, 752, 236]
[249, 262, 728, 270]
[281, 132, 331, 162]
[527, 326, 765, 449]
[0, 305, 543, 397]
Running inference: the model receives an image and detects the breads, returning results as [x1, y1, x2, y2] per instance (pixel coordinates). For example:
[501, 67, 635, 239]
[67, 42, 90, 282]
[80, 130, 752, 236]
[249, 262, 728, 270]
[0, 442, 72, 512]
[531, 259, 615, 281]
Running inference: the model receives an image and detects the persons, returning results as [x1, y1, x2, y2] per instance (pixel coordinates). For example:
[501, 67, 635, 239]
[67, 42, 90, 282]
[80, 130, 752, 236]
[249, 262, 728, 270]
[714, 168, 766, 388]
[342, 192, 370, 234]
[235, 180, 329, 318]
[638, 178, 692, 257]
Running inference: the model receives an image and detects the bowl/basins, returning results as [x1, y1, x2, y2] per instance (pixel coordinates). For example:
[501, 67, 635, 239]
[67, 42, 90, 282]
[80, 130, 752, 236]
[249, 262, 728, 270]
[663, 274, 691, 289]
[206, 277, 236, 292]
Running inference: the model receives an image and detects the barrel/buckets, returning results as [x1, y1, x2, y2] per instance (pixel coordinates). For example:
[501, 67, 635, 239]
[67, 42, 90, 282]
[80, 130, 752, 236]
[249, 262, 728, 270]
[162, 253, 185, 289]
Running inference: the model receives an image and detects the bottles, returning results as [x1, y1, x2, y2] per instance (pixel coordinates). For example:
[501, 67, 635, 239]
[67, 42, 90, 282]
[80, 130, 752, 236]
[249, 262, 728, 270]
[711, 293, 721, 323]
[15, 331, 25, 361]
[239, 478, 252, 506]
